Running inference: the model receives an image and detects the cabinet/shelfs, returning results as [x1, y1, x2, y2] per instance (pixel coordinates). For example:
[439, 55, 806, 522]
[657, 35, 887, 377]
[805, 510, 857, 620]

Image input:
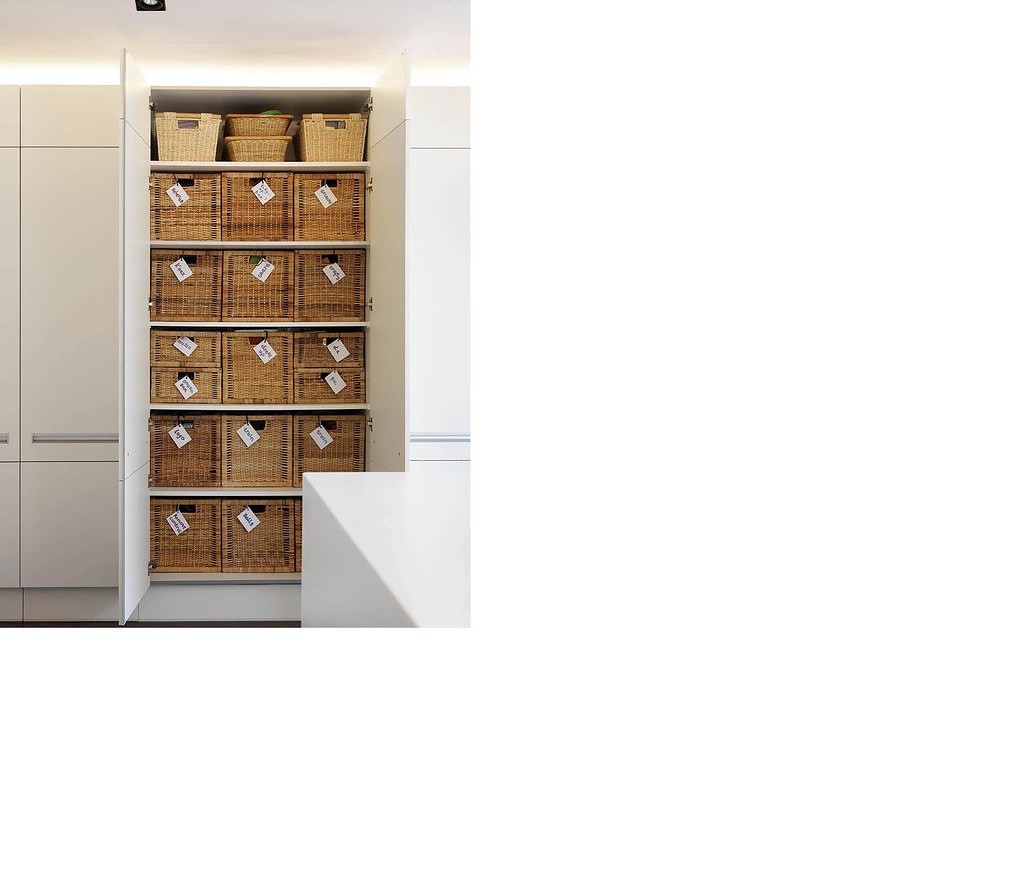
[0, 85, 151, 625]
[120, 50, 407, 624]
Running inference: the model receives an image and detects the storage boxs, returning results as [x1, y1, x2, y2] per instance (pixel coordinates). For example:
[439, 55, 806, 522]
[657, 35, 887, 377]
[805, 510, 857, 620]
[294, 173, 364, 241]
[150, 250, 222, 322]
[154, 112, 222, 162]
[150, 331, 221, 367]
[294, 368, 365, 404]
[294, 250, 366, 321]
[222, 500, 294, 572]
[293, 333, 364, 368]
[150, 415, 221, 486]
[222, 173, 293, 242]
[221, 415, 292, 487]
[222, 332, 293, 403]
[293, 113, 369, 162]
[151, 368, 222, 403]
[225, 114, 294, 136]
[224, 136, 293, 162]
[150, 173, 221, 241]
[294, 416, 365, 487]
[222, 251, 293, 321]
[150, 499, 221, 573]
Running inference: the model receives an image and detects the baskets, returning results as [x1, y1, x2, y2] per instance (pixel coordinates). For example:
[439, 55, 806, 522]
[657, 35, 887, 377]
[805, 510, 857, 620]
[293, 113, 369, 163]
[225, 113, 294, 136]
[221, 134, 291, 162]
[155, 112, 222, 162]
